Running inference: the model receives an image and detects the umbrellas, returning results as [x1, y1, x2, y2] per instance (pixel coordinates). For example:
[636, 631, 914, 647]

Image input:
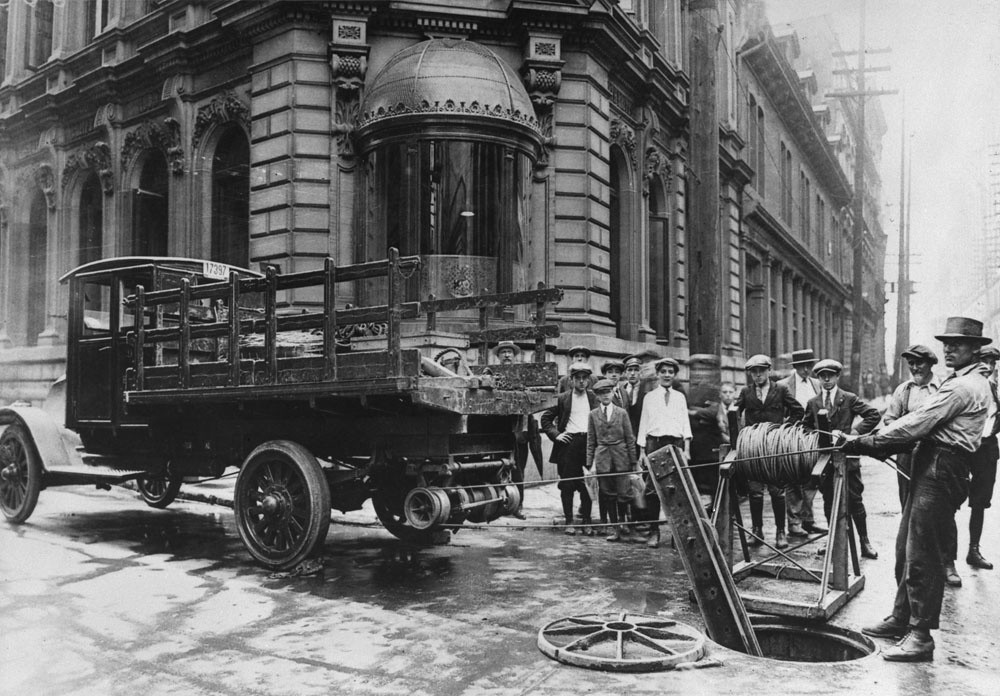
[528, 414, 543, 480]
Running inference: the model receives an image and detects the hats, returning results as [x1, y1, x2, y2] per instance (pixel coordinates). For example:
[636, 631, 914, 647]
[789, 350, 815, 364]
[813, 359, 843, 378]
[901, 345, 938, 365]
[569, 363, 594, 376]
[569, 345, 591, 359]
[934, 318, 993, 346]
[656, 358, 679, 374]
[593, 379, 615, 391]
[980, 347, 1000, 361]
[601, 360, 625, 374]
[493, 340, 520, 357]
[746, 354, 772, 369]
[624, 356, 643, 369]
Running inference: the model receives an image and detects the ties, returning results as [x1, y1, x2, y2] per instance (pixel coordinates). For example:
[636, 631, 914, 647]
[629, 386, 634, 405]
[604, 406, 608, 422]
[665, 387, 670, 407]
[726, 406, 729, 413]
[825, 391, 833, 412]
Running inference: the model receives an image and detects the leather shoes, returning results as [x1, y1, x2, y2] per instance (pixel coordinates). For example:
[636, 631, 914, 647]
[817, 550, 827, 553]
[861, 539, 877, 558]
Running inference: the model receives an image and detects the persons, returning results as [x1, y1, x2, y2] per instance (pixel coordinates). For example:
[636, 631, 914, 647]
[558, 345, 599, 515]
[839, 317, 992, 662]
[729, 355, 805, 549]
[801, 358, 882, 559]
[593, 360, 631, 417]
[688, 380, 746, 451]
[638, 357, 693, 548]
[492, 340, 529, 520]
[883, 344, 941, 516]
[774, 349, 830, 537]
[620, 355, 653, 461]
[584, 379, 648, 543]
[937, 347, 1000, 586]
[540, 362, 600, 537]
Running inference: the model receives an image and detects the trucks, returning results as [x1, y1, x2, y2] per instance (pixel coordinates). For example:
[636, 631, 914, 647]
[0, 246, 565, 572]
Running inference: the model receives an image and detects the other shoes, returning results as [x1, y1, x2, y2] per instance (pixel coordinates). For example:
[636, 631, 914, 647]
[862, 615, 911, 638]
[884, 626, 935, 662]
[565, 512, 829, 547]
[966, 547, 993, 569]
[945, 561, 962, 588]
